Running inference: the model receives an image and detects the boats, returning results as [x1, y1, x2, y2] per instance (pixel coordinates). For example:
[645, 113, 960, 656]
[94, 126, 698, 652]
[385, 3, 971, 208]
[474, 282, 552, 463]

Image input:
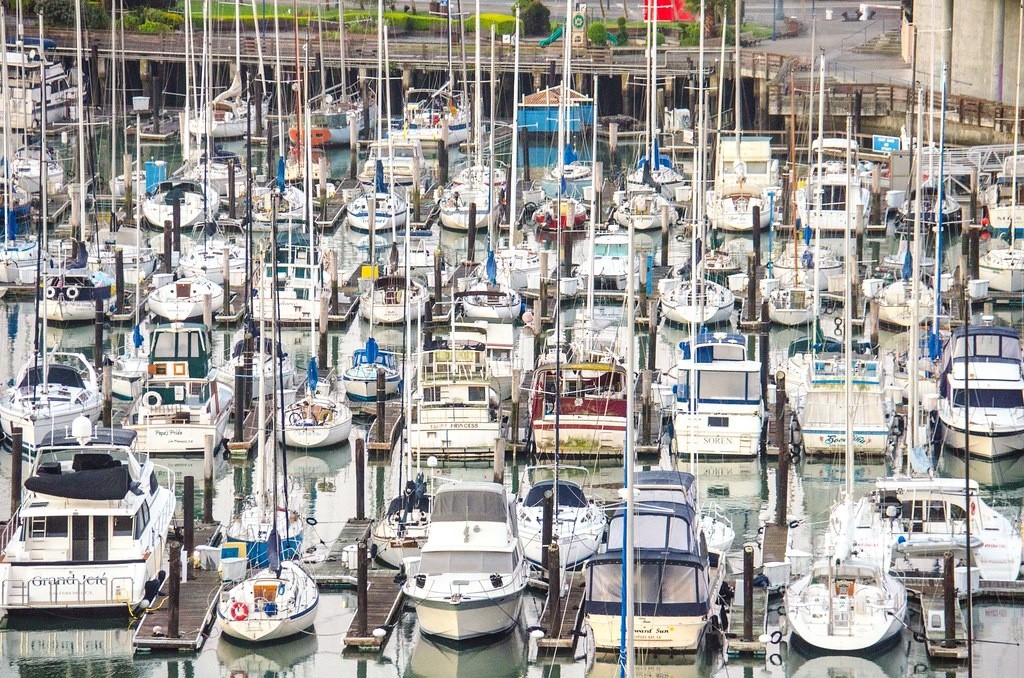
[410, 604, 532, 678]
[784, 629, 910, 678]
[0, 610, 138, 661]
[215, 625, 320, 678]
[584, 646, 716, 678]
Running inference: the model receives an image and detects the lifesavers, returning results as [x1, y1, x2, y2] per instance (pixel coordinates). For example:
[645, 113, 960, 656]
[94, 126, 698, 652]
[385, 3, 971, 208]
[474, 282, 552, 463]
[66, 287, 79, 299]
[770, 630, 782, 645]
[777, 586, 785, 595]
[305, 517, 317, 526]
[319, 409, 333, 423]
[447, 200, 454, 207]
[770, 654, 782, 666]
[914, 664, 927, 674]
[143, 391, 163, 410]
[229, 670, 247, 678]
[912, 632, 926, 643]
[46, 287, 55, 299]
[230, 601, 248, 620]
[777, 606, 786, 616]
[437, 186, 444, 198]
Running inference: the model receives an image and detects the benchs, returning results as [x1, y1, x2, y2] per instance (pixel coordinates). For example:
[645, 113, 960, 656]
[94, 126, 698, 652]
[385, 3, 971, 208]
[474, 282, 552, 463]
[740, 31, 761, 48]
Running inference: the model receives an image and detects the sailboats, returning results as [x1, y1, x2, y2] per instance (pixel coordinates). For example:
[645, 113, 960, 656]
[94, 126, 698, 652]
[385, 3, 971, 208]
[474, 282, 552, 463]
[0, 1, 1023, 653]
